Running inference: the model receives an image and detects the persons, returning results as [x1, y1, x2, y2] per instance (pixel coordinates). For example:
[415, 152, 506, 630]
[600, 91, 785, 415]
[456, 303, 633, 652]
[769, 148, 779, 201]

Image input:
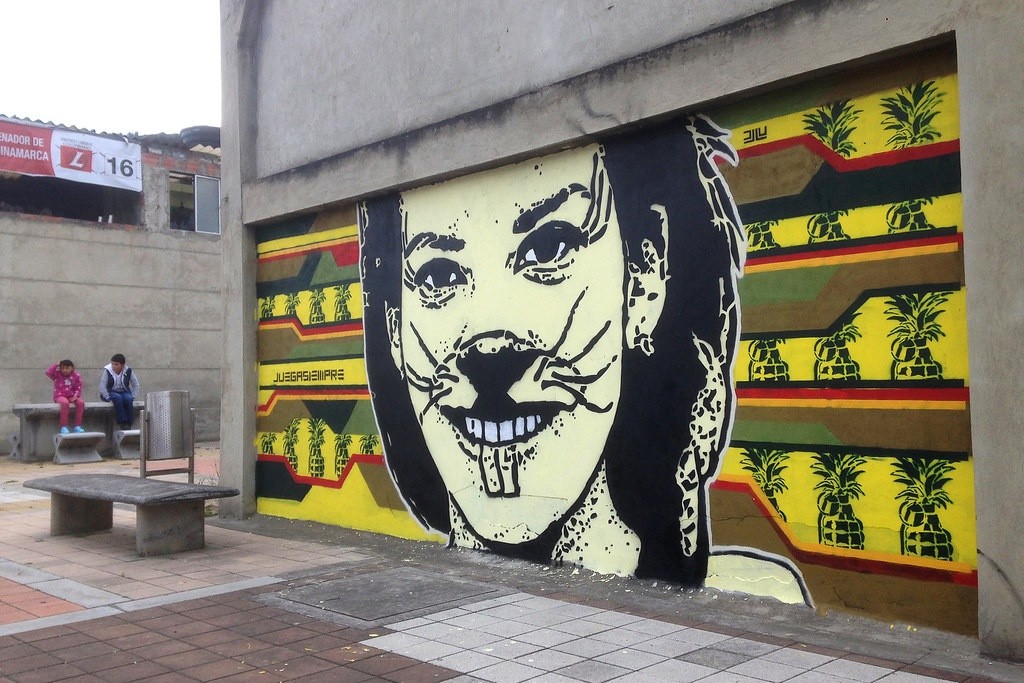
[45, 359, 85, 434]
[100, 353, 139, 430]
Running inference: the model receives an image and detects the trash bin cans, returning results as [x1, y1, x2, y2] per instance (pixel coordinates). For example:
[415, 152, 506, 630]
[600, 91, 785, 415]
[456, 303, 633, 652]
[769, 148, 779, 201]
[140, 390, 194, 482]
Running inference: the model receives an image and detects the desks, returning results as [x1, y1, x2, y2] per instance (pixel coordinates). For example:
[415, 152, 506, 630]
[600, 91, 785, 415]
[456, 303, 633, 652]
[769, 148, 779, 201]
[13, 400, 145, 463]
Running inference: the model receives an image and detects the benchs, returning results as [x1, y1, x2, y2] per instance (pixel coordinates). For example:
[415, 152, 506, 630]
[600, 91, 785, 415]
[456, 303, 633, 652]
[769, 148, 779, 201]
[112, 429, 141, 460]
[8, 433, 22, 460]
[22, 474, 240, 558]
[51, 432, 106, 464]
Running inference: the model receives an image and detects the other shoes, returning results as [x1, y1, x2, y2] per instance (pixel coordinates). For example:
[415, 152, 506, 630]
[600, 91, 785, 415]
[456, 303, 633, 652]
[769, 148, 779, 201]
[60, 426, 69, 434]
[73, 426, 85, 433]
[121, 422, 131, 430]
